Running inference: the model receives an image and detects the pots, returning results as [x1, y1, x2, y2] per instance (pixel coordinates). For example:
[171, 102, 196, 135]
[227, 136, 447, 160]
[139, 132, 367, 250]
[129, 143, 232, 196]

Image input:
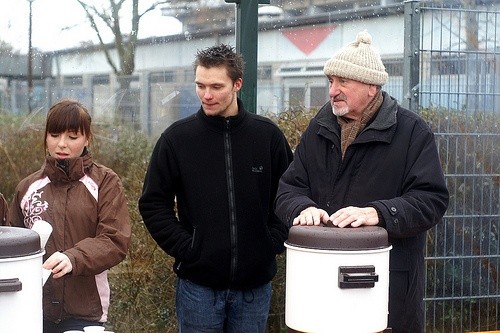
[284, 221, 392, 333]
[0, 226, 46, 333]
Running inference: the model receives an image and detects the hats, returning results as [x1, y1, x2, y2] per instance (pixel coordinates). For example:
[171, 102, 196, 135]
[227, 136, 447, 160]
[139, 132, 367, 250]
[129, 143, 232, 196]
[323, 30, 389, 86]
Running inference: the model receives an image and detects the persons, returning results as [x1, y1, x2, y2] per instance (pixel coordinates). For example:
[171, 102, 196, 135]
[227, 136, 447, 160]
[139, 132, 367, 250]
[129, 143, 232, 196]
[138, 43, 294, 333]
[275, 31, 449, 333]
[10, 101, 132, 333]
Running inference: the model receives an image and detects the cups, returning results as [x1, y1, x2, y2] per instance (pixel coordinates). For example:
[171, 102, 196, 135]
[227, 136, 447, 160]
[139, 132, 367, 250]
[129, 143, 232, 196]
[31, 220, 53, 248]
[83, 326, 105, 333]
